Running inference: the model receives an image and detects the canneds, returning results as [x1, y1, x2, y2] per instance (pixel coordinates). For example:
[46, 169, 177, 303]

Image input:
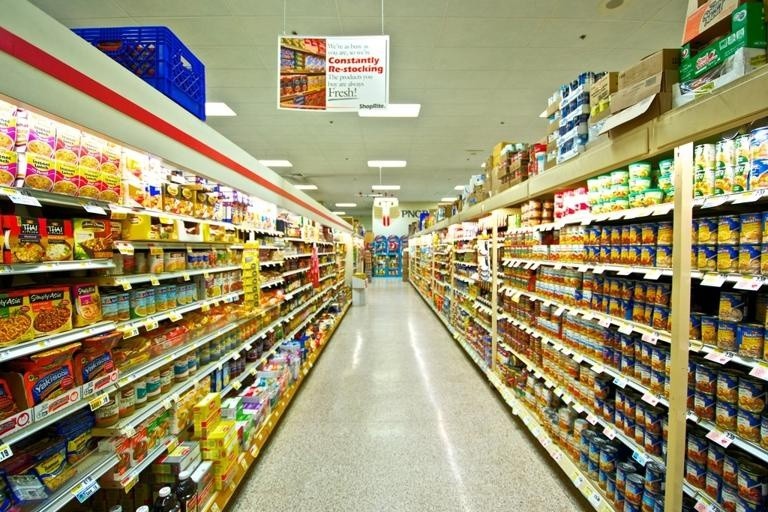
[681, 125, 767, 512]
[536, 159, 675, 512]
[94, 252, 255, 429]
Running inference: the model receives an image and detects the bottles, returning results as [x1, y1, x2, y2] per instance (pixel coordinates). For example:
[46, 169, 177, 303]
[153, 469, 199, 512]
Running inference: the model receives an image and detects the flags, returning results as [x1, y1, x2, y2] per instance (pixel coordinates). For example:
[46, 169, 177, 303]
[275, 33, 391, 114]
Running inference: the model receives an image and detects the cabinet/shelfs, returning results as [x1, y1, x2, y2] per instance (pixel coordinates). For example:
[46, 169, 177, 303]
[365, 231, 407, 278]
[409, 65, 767, 512]
[1, 93, 366, 507]
